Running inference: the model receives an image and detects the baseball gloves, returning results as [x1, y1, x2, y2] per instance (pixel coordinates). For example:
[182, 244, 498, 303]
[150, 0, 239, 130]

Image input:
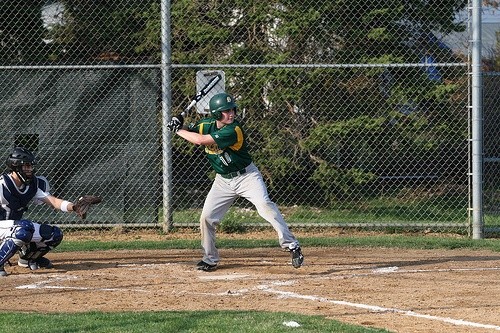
[72, 195, 102, 220]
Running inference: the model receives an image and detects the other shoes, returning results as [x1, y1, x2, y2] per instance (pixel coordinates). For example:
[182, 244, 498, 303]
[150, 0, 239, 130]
[0, 263, 7, 276]
[18, 256, 52, 270]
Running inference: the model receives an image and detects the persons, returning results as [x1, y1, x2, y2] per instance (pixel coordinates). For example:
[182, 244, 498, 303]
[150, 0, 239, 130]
[171, 91, 305, 271]
[0, 148, 102, 276]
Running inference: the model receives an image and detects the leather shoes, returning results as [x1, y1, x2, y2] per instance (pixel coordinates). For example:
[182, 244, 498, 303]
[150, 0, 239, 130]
[220, 169, 247, 180]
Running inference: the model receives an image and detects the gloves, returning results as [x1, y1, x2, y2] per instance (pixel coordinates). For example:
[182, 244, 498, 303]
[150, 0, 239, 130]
[166, 120, 181, 132]
[170, 113, 184, 125]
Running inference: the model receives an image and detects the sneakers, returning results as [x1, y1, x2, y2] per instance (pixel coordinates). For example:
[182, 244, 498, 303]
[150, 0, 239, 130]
[195, 260, 218, 272]
[288, 239, 303, 268]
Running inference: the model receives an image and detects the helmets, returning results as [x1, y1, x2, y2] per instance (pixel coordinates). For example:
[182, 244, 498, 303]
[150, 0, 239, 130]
[5, 147, 38, 185]
[209, 92, 237, 120]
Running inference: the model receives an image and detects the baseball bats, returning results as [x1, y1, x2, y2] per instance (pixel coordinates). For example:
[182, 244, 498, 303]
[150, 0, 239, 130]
[166, 73, 222, 132]
